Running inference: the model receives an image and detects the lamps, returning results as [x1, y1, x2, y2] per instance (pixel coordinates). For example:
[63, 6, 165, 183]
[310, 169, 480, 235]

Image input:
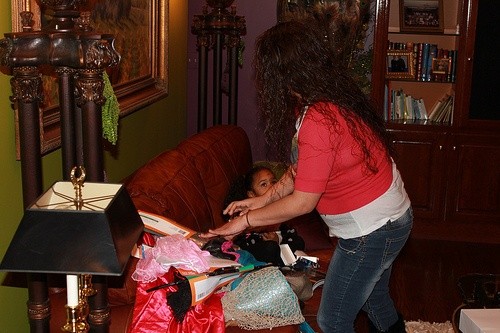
[0, 165, 144, 332]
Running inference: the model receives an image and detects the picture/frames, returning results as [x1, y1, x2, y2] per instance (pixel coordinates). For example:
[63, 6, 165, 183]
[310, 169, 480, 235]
[385, 49, 416, 79]
[431, 56, 452, 74]
[10, 0, 169, 162]
[399, 0, 444, 33]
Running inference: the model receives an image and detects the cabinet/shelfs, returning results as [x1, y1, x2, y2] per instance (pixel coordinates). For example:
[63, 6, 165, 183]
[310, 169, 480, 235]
[371, 0, 500, 222]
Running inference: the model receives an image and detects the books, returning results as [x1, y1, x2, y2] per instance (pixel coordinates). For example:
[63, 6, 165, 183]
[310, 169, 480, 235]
[413, 41, 457, 82]
[385, 84, 454, 123]
[388, 41, 412, 49]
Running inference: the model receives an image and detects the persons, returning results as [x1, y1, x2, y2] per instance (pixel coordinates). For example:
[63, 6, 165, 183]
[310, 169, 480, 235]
[405, 11, 439, 25]
[233, 167, 312, 307]
[391, 55, 405, 71]
[199, 22, 412, 333]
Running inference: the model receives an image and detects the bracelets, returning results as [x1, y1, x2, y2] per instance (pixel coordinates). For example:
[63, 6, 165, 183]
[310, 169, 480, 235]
[246, 210, 253, 228]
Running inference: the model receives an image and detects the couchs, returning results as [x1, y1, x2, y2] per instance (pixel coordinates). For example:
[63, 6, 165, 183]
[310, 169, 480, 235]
[48, 125, 333, 332]
